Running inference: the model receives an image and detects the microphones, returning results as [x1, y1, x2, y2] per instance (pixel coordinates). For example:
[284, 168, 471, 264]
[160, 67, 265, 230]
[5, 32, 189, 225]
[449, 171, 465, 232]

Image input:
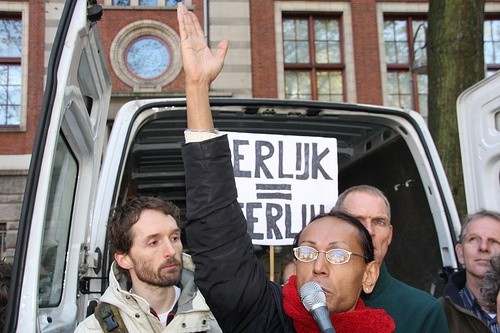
[300, 281, 335, 333]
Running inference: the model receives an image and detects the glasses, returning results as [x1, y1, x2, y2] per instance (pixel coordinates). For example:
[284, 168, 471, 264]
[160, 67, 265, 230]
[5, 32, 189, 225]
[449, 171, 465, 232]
[292, 246, 369, 265]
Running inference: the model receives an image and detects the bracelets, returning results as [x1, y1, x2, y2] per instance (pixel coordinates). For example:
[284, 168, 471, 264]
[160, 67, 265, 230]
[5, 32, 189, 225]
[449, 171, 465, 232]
[185, 127, 219, 138]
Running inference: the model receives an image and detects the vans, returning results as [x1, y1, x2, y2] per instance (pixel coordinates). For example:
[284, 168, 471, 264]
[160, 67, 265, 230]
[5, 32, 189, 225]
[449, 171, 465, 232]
[4, 0, 500, 333]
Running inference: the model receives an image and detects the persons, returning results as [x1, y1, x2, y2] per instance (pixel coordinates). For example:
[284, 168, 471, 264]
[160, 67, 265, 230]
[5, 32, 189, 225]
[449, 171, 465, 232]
[177, 2, 398, 333]
[74, 195, 225, 333]
[436, 210, 500, 333]
[478, 254, 500, 333]
[334, 185, 449, 333]
